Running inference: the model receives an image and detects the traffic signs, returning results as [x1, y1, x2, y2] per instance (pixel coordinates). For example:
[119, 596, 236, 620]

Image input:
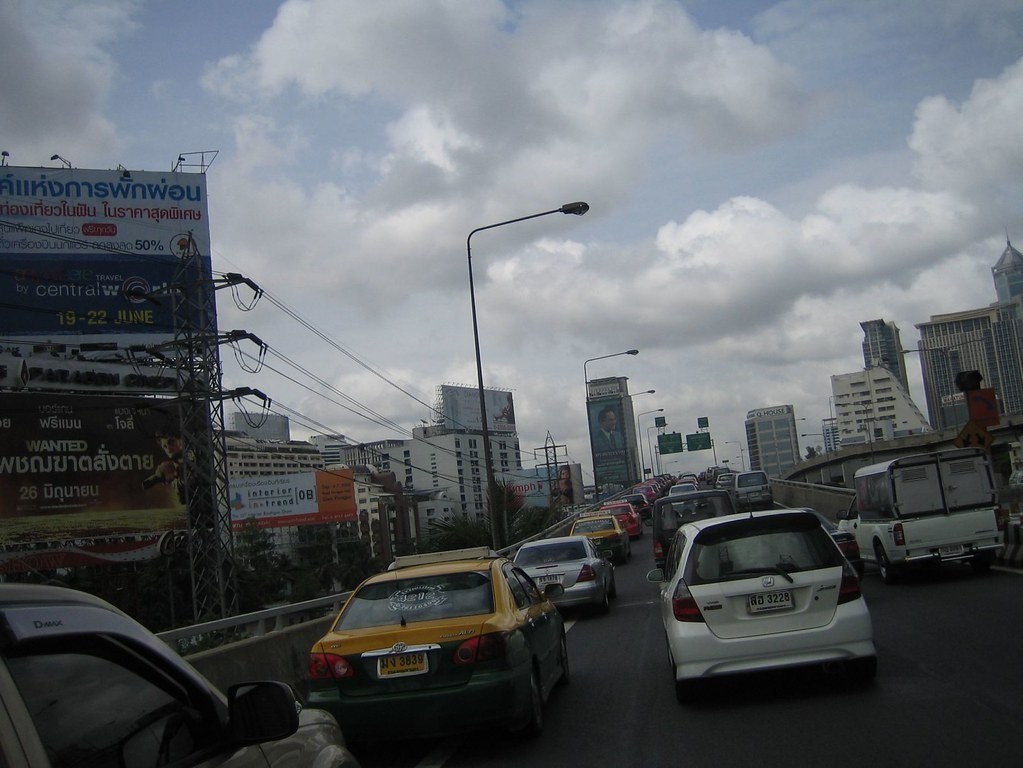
[656, 432, 684, 455]
[685, 431, 712, 452]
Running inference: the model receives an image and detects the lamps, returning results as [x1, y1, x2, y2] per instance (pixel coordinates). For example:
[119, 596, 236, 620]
[2, 151, 9, 166]
[119, 164, 131, 178]
[172, 157, 185, 172]
[51, 154, 71, 170]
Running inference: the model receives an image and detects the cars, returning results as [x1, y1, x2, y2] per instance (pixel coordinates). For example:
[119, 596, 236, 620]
[512, 534, 619, 616]
[307, 545, 573, 748]
[0, 582, 359, 768]
[780, 506, 865, 584]
[646, 507, 880, 706]
[597, 463, 774, 575]
[569, 509, 633, 567]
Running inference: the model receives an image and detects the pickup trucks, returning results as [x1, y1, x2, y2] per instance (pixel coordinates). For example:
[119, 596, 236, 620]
[834, 444, 1007, 586]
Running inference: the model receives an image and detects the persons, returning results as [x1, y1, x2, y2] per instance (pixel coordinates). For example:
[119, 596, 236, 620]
[552, 466, 572, 504]
[154, 426, 213, 531]
[594, 409, 625, 466]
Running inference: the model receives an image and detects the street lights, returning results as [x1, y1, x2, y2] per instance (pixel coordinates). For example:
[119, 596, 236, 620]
[583, 349, 641, 501]
[793, 418, 806, 463]
[834, 397, 896, 464]
[462, 201, 592, 561]
[647, 423, 669, 478]
[898, 336, 986, 436]
[828, 393, 846, 451]
[724, 440, 745, 472]
[619, 388, 655, 487]
[801, 428, 849, 482]
[637, 408, 665, 481]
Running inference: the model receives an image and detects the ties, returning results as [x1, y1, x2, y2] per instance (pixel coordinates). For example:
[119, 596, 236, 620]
[610, 434, 615, 446]
